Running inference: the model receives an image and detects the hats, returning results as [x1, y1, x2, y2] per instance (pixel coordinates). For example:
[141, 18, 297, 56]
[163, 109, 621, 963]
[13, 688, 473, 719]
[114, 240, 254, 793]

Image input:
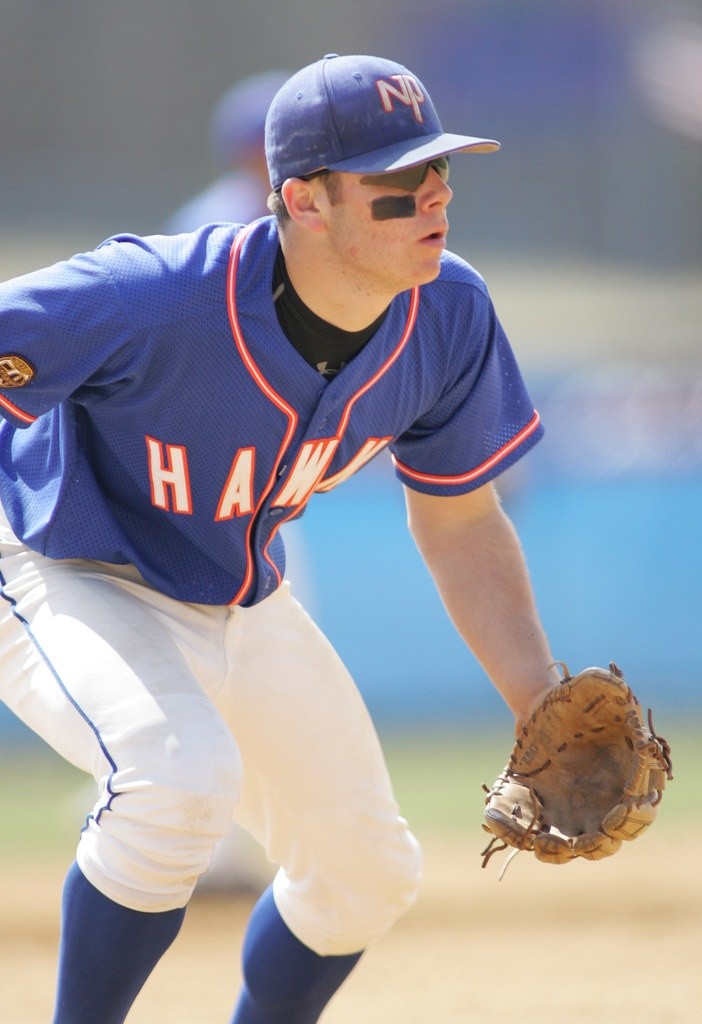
[264, 53, 500, 193]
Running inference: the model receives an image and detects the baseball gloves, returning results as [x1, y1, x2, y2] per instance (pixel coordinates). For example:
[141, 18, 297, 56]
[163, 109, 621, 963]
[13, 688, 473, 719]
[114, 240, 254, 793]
[478, 656, 675, 884]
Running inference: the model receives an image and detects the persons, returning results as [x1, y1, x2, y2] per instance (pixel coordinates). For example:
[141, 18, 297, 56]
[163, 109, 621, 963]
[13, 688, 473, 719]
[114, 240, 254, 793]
[161, 72, 291, 236]
[0, 50, 673, 1024]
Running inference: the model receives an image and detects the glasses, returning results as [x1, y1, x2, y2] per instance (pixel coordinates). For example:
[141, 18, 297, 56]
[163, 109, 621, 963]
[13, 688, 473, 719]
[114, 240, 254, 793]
[274, 154, 450, 195]
